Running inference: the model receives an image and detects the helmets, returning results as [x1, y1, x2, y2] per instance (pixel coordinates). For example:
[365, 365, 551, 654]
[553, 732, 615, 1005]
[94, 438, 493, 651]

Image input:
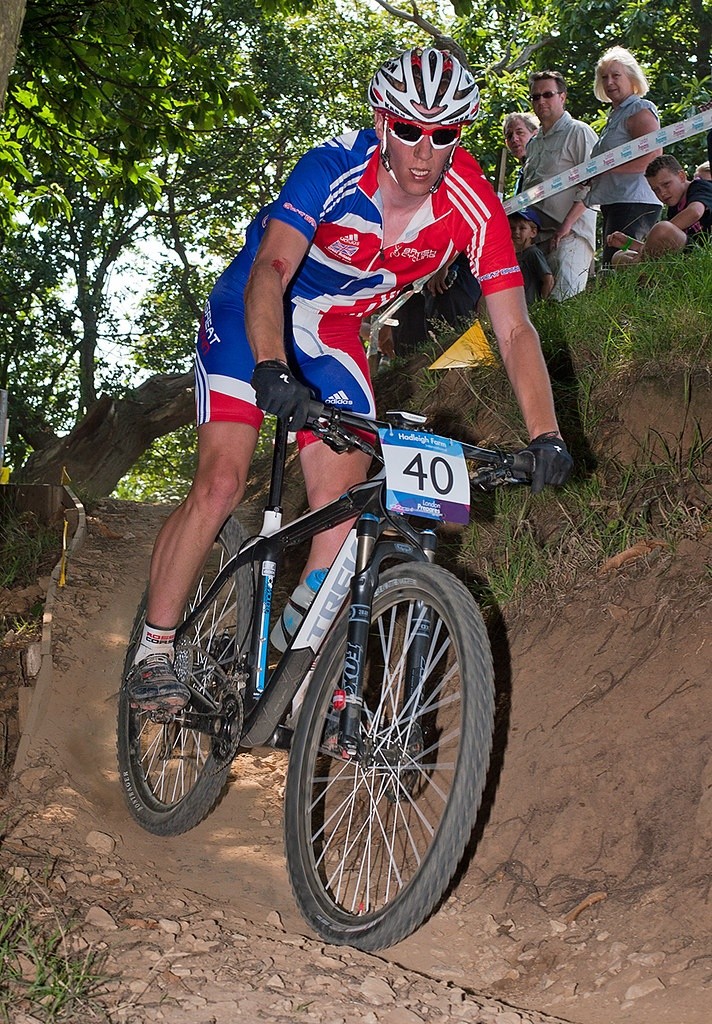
[367, 46, 480, 126]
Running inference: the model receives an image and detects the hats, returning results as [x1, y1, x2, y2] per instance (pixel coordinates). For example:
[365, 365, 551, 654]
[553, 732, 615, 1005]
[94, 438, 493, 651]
[510, 209, 541, 230]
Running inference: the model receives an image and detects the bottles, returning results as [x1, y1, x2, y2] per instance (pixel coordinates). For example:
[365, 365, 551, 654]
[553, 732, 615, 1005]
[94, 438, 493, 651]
[270, 567, 329, 653]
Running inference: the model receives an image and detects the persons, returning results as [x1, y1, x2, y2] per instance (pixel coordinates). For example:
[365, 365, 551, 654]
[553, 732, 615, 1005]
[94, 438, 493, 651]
[505, 209, 556, 312]
[502, 112, 541, 199]
[358, 253, 481, 370]
[124, 44, 577, 715]
[693, 161, 712, 180]
[606, 154, 712, 269]
[578, 45, 664, 271]
[521, 70, 601, 303]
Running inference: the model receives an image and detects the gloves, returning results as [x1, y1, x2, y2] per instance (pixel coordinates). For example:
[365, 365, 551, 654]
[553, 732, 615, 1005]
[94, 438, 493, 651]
[250, 360, 313, 431]
[513, 433, 573, 494]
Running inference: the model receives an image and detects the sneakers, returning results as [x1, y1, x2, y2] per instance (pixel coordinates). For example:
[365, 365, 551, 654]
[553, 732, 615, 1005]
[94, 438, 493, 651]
[323, 707, 375, 746]
[125, 652, 191, 714]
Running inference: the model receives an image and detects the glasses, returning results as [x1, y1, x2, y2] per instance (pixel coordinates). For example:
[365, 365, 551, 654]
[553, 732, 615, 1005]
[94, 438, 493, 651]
[530, 90, 563, 101]
[383, 115, 462, 150]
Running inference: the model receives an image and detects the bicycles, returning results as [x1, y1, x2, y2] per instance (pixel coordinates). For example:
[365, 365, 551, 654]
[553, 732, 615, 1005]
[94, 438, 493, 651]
[117, 390, 565, 953]
